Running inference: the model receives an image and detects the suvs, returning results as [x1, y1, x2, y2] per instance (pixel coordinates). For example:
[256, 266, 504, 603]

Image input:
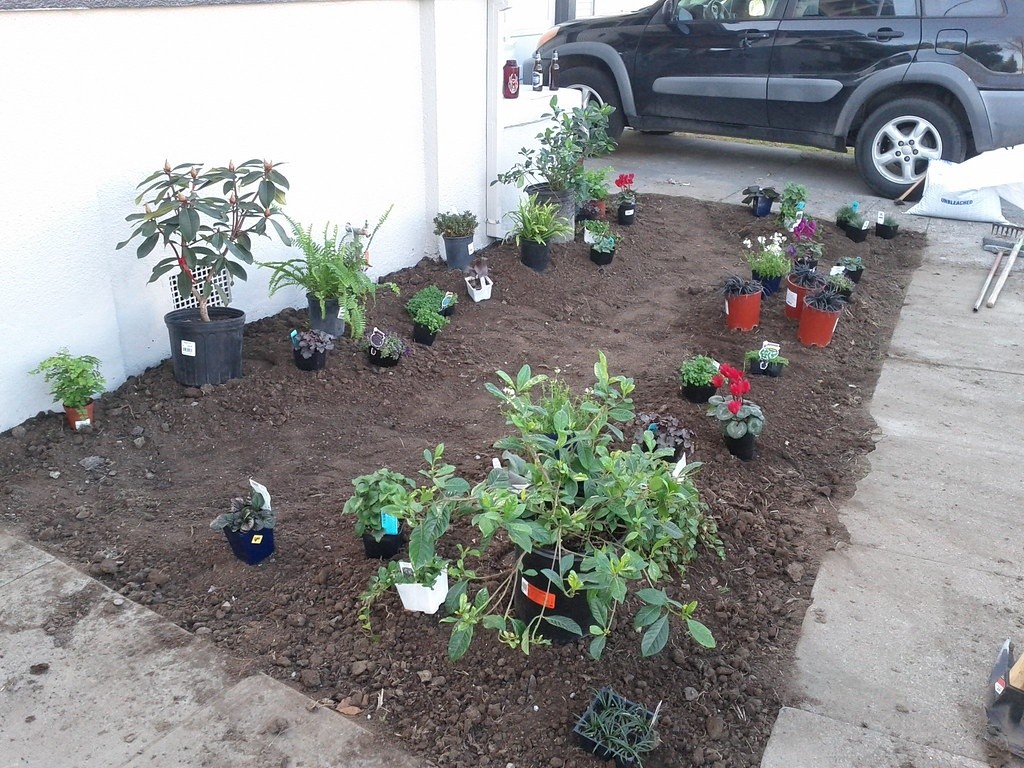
[522, 0, 1024, 200]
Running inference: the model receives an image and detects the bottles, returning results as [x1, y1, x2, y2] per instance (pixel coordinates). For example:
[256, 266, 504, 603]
[503, 60, 520, 98]
[549, 50, 560, 90]
[532, 50, 543, 91]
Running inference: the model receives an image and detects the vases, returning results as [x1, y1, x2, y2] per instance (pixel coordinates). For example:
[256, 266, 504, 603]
[750, 271, 781, 297]
[795, 259, 819, 271]
[750, 360, 783, 376]
[618, 204, 635, 225]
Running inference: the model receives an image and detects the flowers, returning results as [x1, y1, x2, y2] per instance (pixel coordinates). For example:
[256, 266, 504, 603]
[742, 231, 788, 278]
[616, 173, 640, 205]
[786, 213, 826, 261]
[705, 362, 763, 440]
[745, 341, 791, 367]
[825, 265, 856, 293]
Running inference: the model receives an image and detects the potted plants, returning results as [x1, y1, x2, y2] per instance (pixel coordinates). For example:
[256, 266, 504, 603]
[571, 686, 663, 767]
[722, 256, 867, 348]
[726, 433, 755, 461]
[116, 96, 725, 661]
[28, 349, 104, 429]
[779, 181, 807, 233]
[742, 186, 780, 218]
[835, 204, 899, 243]
